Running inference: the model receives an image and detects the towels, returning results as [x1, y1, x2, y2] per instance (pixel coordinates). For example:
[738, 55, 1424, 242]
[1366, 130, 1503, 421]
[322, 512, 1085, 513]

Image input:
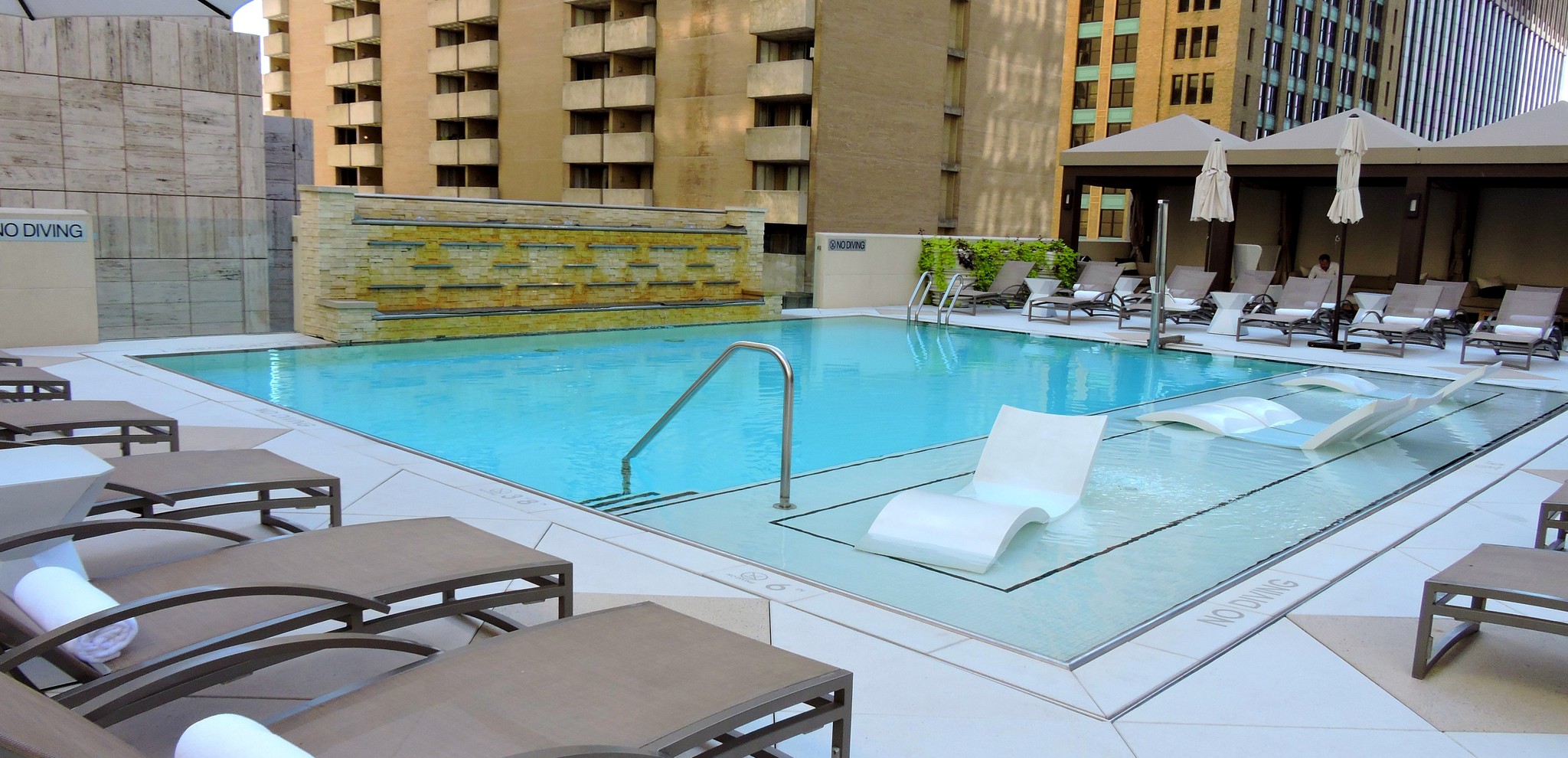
[1303, 301, 1337, 310]
[1382, 315, 1426, 327]
[1509, 314, 1551, 328]
[1073, 284, 1096, 291]
[1275, 308, 1314, 317]
[1494, 324, 1543, 337]
[1170, 289, 1186, 298]
[1074, 290, 1104, 301]
[173, 712, 317, 758]
[1170, 298, 1197, 306]
[11, 566, 139, 664]
[1413, 308, 1451, 318]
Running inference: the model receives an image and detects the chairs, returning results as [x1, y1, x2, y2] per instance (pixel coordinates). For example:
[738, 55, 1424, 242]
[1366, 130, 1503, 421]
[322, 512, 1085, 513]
[1535, 480, 1567, 553]
[1192, 391, 1447, 442]
[1136, 394, 1408, 453]
[0, 517, 572, 715]
[1276, 356, 1505, 412]
[854, 404, 1108, 577]
[1413, 542, 1568, 674]
[0, 367, 75, 438]
[0, 350, 21, 366]
[956, 259, 1566, 373]
[0, 400, 180, 457]
[42, 449, 344, 555]
[0, 601, 851, 758]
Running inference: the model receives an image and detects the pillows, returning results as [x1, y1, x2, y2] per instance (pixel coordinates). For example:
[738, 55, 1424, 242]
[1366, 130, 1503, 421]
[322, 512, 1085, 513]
[1476, 275, 1505, 297]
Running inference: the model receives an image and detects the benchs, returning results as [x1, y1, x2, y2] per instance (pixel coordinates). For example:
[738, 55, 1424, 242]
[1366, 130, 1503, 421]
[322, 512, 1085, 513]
[1460, 274, 1567, 330]
[1343, 274, 1438, 310]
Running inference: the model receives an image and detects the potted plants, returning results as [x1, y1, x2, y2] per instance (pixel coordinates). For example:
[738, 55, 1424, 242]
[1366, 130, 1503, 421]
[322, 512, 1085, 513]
[920, 236, 977, 308]
[1018, 240, 1079, 301]
[971, 239, 1023, 306]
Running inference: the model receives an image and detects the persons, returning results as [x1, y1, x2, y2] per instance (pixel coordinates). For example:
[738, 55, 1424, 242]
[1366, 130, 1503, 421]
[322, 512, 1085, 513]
[1307, 254, 1338, 279]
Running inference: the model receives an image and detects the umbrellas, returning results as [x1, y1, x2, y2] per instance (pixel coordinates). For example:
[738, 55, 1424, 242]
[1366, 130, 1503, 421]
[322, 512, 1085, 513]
[1326, 114, 1363, 341]
[1188, 140, 1234, 272]
